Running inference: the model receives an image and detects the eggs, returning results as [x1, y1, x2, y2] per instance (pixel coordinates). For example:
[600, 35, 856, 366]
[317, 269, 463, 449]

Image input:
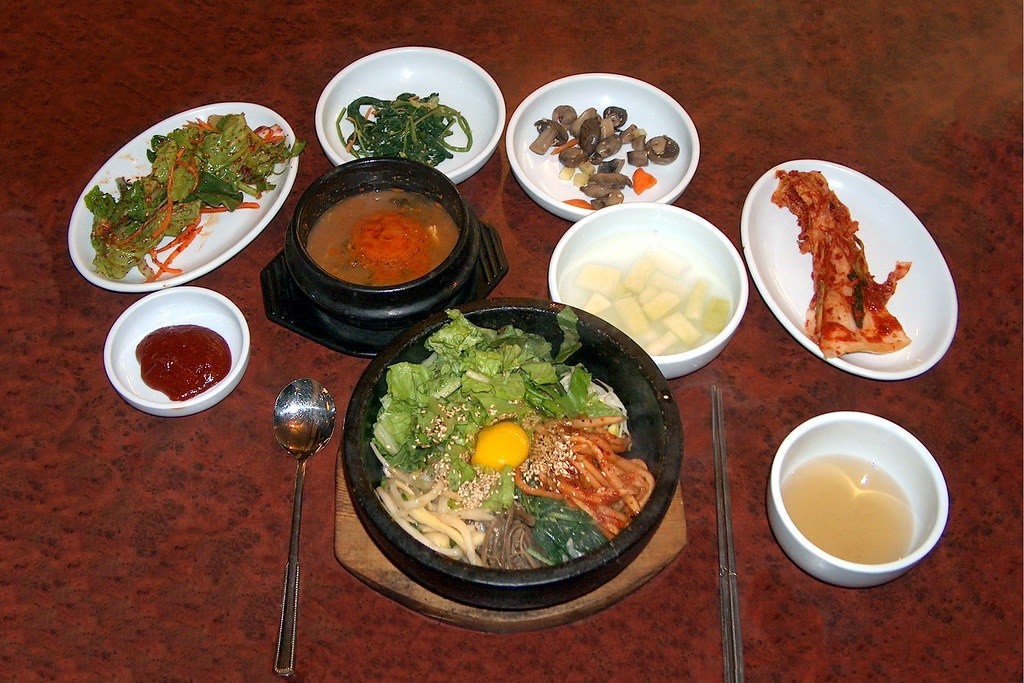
[470, 419, 532, 473]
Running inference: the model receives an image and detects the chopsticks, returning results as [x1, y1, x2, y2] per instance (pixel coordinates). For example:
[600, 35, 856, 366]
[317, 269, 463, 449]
[710, 385, 745, 683]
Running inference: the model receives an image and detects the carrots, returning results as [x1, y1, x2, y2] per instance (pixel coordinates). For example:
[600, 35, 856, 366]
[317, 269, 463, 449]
[632, 168, 657, 195]
[563, 199, 594, 209]
[551, 139, 578, 155]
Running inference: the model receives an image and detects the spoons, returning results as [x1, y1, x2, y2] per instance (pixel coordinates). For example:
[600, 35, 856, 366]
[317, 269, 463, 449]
[272, 377, 337, 678]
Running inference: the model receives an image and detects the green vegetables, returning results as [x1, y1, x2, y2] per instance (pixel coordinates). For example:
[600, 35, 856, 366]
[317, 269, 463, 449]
[336, 93, 473, 167]
[370, 308, 626, 565]
[84, 113, 306, 281]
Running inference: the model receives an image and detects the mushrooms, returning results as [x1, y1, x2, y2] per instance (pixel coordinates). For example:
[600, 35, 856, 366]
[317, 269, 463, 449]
[529, 105, 680, 210]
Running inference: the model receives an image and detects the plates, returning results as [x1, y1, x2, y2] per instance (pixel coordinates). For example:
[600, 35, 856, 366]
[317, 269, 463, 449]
[741, 158, 958, 381]
[68, 101, 299, 292]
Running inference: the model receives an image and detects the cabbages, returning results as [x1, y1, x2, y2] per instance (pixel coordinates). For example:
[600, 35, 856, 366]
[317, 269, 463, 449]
[771, 170, 912, 359]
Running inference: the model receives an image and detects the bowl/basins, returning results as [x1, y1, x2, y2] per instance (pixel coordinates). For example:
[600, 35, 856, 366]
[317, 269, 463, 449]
[767, 410, 948, 587]
[505, 73, 700, 222]
[283, 157, 481, 330]
[103, 286, 251, 418]
[342, 296, 683, 610]
[548, 202, 749, 381]
[315, 46, 506, 185]
